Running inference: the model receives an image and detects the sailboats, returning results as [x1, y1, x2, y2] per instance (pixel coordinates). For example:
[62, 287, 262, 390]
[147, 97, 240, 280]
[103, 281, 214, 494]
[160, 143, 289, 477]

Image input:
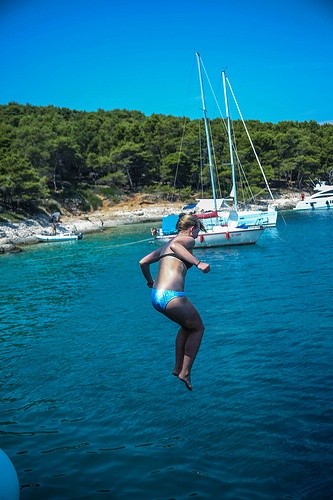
[212, 69, 278, 229]
[155, 50, 264, 248]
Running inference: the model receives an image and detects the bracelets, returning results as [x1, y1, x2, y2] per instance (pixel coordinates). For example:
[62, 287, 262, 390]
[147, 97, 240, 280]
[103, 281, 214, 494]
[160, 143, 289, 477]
[196, 261, 201, 267]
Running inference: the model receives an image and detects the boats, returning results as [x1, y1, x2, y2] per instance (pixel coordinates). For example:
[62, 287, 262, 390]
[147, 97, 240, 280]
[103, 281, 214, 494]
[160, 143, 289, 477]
[36, 225, 82, 242]
[293, 187, 333, 211]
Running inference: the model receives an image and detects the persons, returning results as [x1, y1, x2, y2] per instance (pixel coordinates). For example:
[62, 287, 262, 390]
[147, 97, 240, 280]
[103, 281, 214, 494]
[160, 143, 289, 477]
[100, 215, 105, 231]
[162, 207, 165, 215]
[51, 222, 56, 235]
[299, 190, 305, 201]
[71, 225, 76, 235]
[78, 233, 83, 240]
[151, 228, 156, 236]
[139, 212, 211, 392]
